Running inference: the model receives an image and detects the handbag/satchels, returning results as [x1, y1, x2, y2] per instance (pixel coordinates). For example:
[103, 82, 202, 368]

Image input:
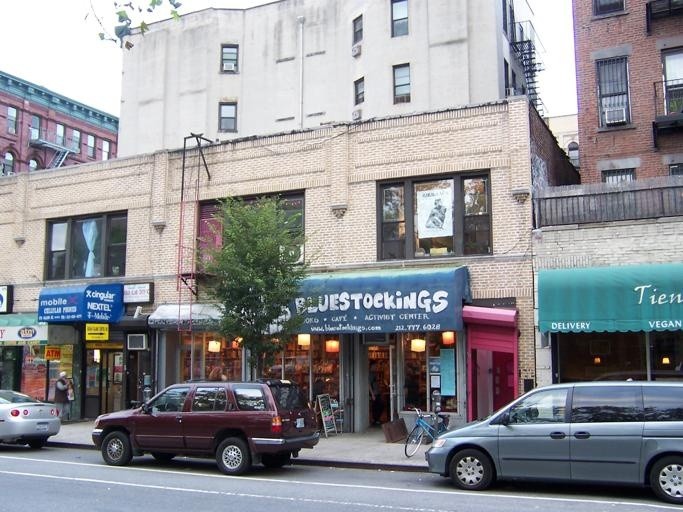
[66, 387, 75, 400]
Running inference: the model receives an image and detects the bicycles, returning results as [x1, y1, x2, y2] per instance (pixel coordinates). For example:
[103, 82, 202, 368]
[405, 406, 450, 459]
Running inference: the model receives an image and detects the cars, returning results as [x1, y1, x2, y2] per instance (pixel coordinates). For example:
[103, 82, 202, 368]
[0, 389, 61, 448]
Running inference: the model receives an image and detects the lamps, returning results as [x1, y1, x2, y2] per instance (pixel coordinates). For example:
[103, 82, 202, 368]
[294, 331, 339, 353]
[207, 332, 241, 354]
[411, 328, 455, 353]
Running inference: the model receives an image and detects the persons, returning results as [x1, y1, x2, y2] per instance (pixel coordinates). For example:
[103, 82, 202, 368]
[405, 364, 420, 408]
[368, 361, 384, 424]
[54, 371, 71, 418]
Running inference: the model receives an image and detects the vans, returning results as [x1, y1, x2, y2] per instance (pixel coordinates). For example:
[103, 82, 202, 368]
[588, 370, 683, 382]
[422, 381, 682, 504]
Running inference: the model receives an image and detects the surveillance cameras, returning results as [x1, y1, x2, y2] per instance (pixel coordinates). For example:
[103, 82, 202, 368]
[134, 306, 142, 319]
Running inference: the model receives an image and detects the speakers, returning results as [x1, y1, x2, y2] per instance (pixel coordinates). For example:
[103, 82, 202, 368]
[128, 334, 148, 350]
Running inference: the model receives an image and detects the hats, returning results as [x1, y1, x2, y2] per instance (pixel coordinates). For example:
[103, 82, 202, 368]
[60, 371, 66, 377]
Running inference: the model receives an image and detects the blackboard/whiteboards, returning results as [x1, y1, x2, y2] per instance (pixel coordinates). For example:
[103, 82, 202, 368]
[318, 394, 336, 432]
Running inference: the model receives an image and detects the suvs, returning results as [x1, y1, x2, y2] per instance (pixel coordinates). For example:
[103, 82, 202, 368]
[89, 378, 320, 476]
